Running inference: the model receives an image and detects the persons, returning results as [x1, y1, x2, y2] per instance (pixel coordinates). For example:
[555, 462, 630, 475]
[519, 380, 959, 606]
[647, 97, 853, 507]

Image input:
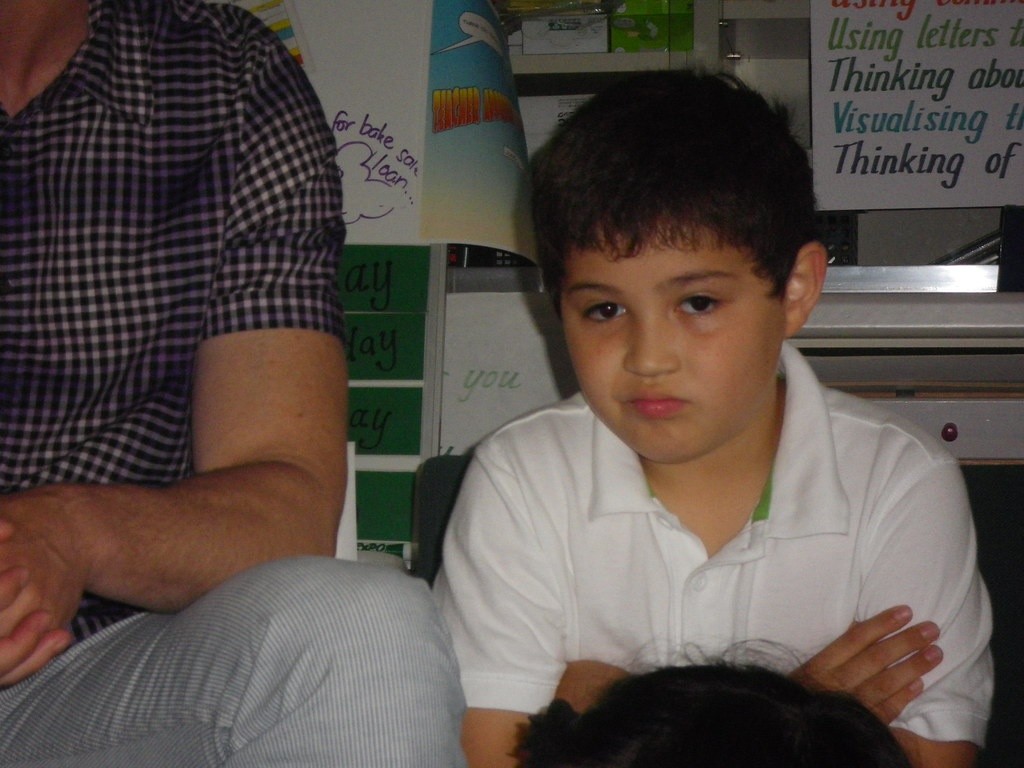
[0, 0, 468, 768]
[525, 664, 913, 767]
[434, 73, 995, 767]
